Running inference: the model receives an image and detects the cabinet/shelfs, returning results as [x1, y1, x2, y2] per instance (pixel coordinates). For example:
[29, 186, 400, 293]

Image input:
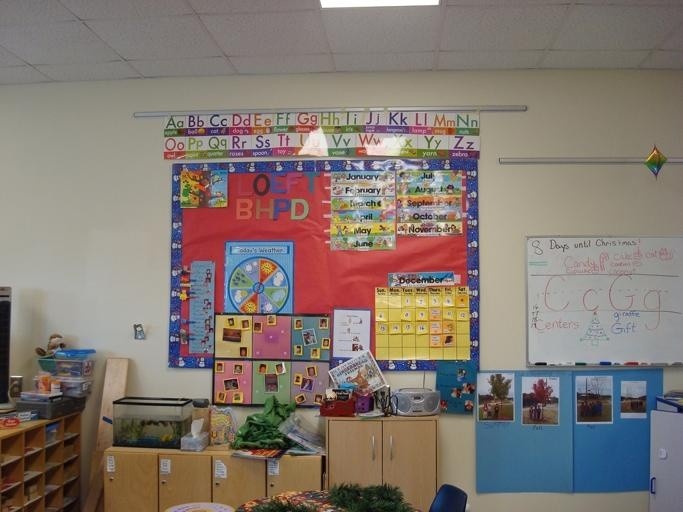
[649, 395, 683, 512]
[323, 416, 439, 512]
[103, 444, 321, 512]
[1, 411, 82, 512]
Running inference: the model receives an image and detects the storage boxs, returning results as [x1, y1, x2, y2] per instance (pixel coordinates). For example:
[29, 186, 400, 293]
[32, 348, 96, 399]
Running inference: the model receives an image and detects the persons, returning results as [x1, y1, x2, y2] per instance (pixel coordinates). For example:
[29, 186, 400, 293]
[439, 370, 643, 423]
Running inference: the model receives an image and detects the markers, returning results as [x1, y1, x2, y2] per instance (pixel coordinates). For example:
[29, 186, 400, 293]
[527, 362, 682, 366]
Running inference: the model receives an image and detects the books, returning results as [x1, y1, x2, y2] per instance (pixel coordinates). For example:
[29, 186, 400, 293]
[230, 425, 326, 461]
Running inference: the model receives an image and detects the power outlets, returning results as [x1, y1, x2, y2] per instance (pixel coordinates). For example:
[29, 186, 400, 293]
[9, 376, 24, 398]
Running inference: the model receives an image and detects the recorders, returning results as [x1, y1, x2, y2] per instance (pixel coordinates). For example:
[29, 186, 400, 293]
[389, 387, 441, 417]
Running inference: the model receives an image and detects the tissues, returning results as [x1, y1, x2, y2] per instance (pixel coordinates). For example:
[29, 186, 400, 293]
[181, 418, 209, 452]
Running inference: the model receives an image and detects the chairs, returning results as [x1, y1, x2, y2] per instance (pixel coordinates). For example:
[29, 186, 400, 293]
[429, 483, 468, 512]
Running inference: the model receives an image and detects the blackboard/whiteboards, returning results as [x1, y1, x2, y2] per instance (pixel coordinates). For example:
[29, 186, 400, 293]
[526, 234, 683, 368]
